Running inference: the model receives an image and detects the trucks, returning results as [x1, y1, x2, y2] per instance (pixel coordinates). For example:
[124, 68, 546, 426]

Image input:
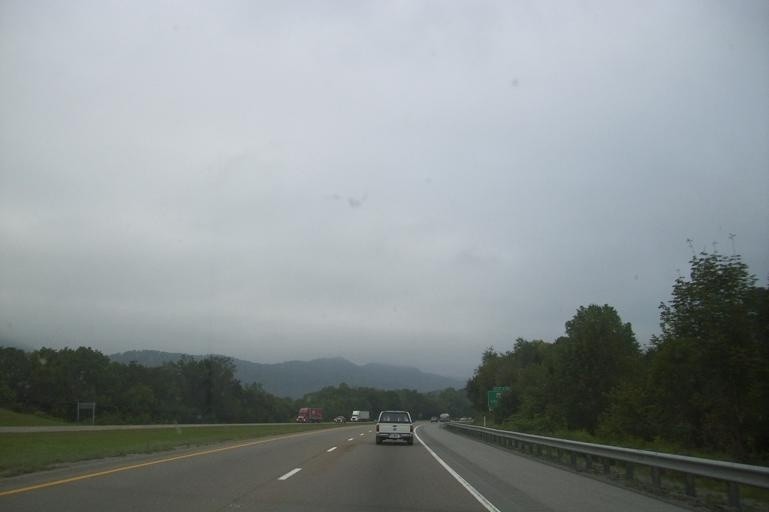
[351, 410, 370, 422]
[440, 413, 450, 423]
[296, 408, 322, 423]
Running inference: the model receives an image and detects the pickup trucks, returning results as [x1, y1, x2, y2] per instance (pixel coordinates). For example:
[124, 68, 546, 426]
[375, 411, 414, 446]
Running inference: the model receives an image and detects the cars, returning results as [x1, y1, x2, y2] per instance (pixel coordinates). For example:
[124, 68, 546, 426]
[459, 417, 472, 422]
[333, 416, 346, 424]
[430, 417, 439, 423]
